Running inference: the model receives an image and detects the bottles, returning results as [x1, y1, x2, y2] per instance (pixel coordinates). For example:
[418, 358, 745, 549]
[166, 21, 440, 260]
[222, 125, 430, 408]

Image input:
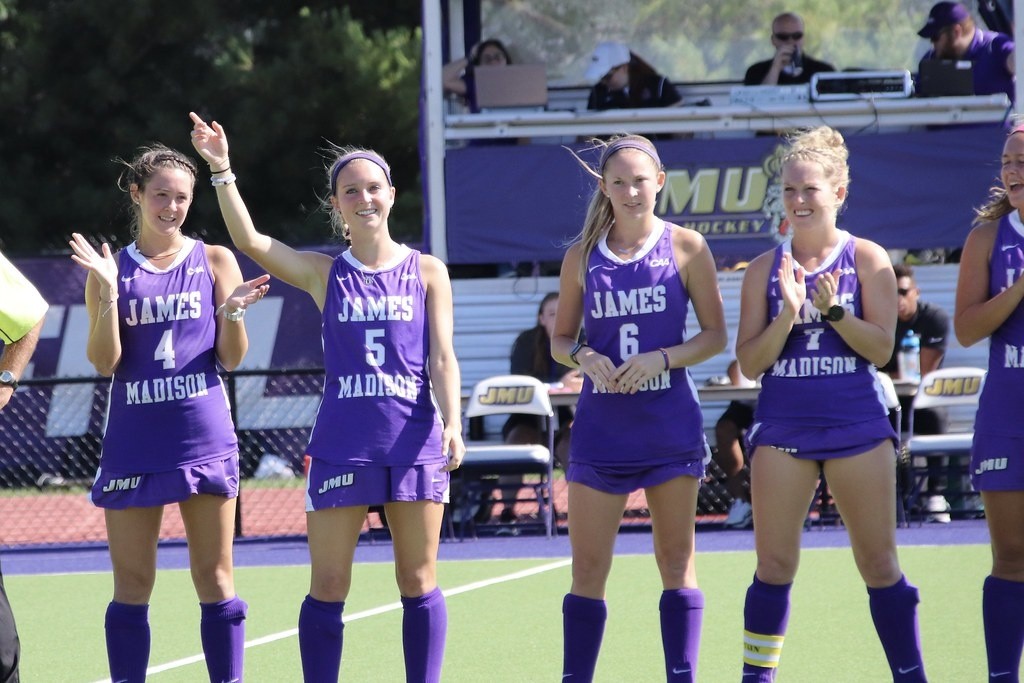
[790, 44, 802, 75]
[901, 330, 921, 380]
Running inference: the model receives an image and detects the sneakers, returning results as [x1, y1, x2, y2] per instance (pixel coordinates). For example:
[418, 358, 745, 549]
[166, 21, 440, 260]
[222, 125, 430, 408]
[496, 509, 521, 536]
[724, 496, 754, 529]
[926, 494, 950, 523]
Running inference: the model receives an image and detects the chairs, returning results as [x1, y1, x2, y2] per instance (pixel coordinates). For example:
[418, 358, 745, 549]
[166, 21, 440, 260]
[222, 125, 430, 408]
[442, 375, 558, 544]
[804, 372, 902, 532]
[896, 366, 987, 529]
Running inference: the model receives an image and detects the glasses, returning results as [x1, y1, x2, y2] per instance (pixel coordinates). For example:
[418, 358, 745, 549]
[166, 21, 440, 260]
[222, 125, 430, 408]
[482, 53, 505, 63]
[774, 32, 803, 42]
[897, 287, 912, 297]
[931, 25, 961, 41]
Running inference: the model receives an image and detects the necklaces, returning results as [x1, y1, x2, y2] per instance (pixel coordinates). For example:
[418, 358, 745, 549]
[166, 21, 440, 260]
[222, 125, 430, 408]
[135, 245, 182, 257]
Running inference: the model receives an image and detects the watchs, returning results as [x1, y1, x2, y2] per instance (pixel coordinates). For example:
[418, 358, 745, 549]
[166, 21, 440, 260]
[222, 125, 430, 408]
[549, 132, 730, 683]
[216, 303, 246, 321]
[820, 304, 845, 321]
[0, 370, 18, 390]
[569, 343, 590, 364]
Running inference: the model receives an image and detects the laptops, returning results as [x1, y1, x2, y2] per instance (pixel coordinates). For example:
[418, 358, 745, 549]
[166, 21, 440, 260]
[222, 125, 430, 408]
[474, 63, 548, 110]
[918, 59, 974, 98]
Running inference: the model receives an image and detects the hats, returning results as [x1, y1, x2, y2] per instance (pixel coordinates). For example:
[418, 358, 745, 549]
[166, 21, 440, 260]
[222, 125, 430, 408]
[584, 43, 631, 87]
[918, 3, 968, 38]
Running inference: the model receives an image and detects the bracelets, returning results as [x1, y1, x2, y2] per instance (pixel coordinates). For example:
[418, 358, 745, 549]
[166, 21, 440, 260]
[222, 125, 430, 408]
[211, 166, 231, 174]
[656, 347, 669, 371]
[98, 293, 120, 317]
[207, 157, 229, 165]
[211, 173, 235, 182]
[212, 177, 237, 186]
[466, 56, 472, 65]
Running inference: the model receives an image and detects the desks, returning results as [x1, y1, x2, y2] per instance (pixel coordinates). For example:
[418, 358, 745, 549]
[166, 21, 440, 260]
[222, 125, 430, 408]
[461, 379, 920, 537]
[444, 93, 1012, 151]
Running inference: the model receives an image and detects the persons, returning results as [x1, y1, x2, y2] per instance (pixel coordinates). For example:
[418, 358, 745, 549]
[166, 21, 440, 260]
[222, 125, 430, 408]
[189, 112, 466, 683]
[443, 37, 532, 279]
[496, 260, 953, 536]
[735, 124, 931, 683]
[912, 1, 1017, 129]
[954, 123, 1024, 683]
[743, 11, 837, 137]
[70, 142, 271, 683]
[0, 250, 48, 683]
[578, 42, 694, 142]
